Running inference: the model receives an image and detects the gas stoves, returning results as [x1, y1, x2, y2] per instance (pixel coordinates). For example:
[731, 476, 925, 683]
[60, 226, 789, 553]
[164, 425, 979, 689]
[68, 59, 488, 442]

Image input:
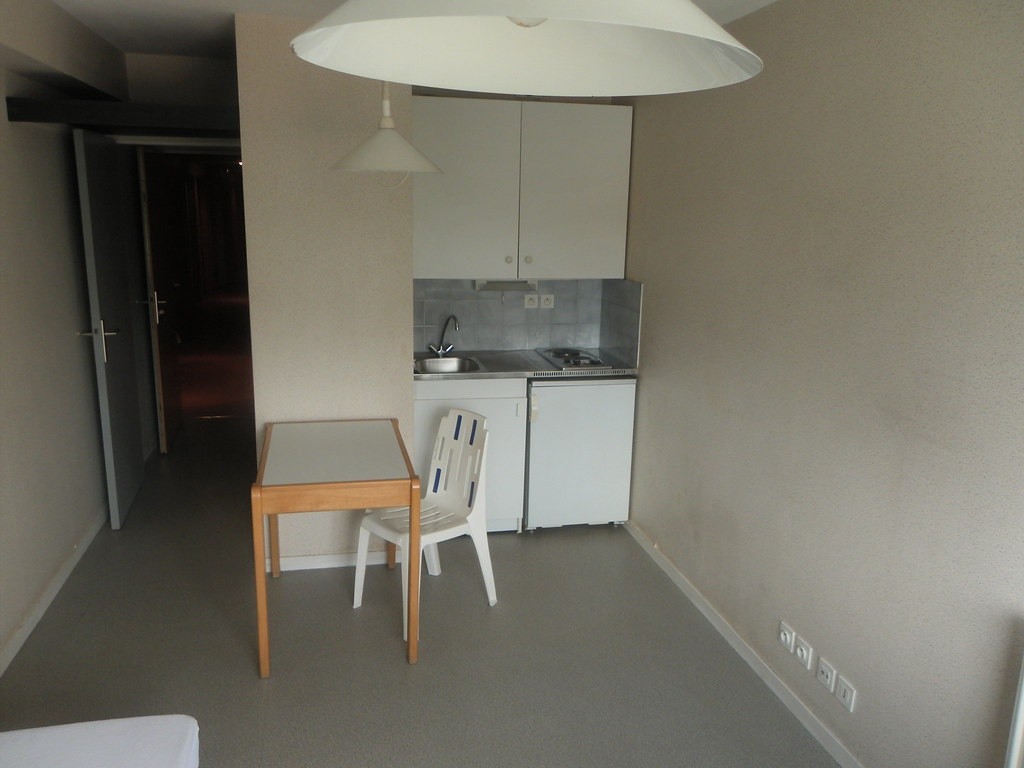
[534, 347, 613, 370]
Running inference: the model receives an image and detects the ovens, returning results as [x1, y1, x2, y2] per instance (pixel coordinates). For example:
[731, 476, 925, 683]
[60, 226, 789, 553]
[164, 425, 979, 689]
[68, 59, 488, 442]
[525, 375, 637, 526]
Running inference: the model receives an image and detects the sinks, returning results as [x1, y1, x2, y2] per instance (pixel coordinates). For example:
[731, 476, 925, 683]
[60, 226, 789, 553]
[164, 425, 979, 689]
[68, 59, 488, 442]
[414, 354, 489, 374]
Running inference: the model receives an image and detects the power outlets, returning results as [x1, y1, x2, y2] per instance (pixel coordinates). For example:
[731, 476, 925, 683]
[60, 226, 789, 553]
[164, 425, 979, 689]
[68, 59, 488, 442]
[541, 295, 555, 309]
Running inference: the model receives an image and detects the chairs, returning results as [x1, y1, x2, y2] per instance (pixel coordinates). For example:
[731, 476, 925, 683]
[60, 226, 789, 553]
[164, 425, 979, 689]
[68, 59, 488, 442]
[352, 409, 498, 642]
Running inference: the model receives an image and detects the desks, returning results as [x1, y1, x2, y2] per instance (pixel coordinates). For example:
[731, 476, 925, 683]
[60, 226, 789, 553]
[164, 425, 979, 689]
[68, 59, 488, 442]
[249, 418, 421, 680]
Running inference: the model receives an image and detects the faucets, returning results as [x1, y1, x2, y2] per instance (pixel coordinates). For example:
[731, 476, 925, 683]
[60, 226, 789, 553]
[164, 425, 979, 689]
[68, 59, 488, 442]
[426, 314, 459, 358]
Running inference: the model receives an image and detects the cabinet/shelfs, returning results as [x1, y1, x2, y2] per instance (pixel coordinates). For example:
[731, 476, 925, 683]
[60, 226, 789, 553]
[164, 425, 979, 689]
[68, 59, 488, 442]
[408, 379, 530, 534]
[406, 96, 633, 282]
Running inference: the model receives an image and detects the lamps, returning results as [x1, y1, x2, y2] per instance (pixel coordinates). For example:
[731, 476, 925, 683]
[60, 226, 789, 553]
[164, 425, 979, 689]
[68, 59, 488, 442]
[287, 1, 763, 99]
